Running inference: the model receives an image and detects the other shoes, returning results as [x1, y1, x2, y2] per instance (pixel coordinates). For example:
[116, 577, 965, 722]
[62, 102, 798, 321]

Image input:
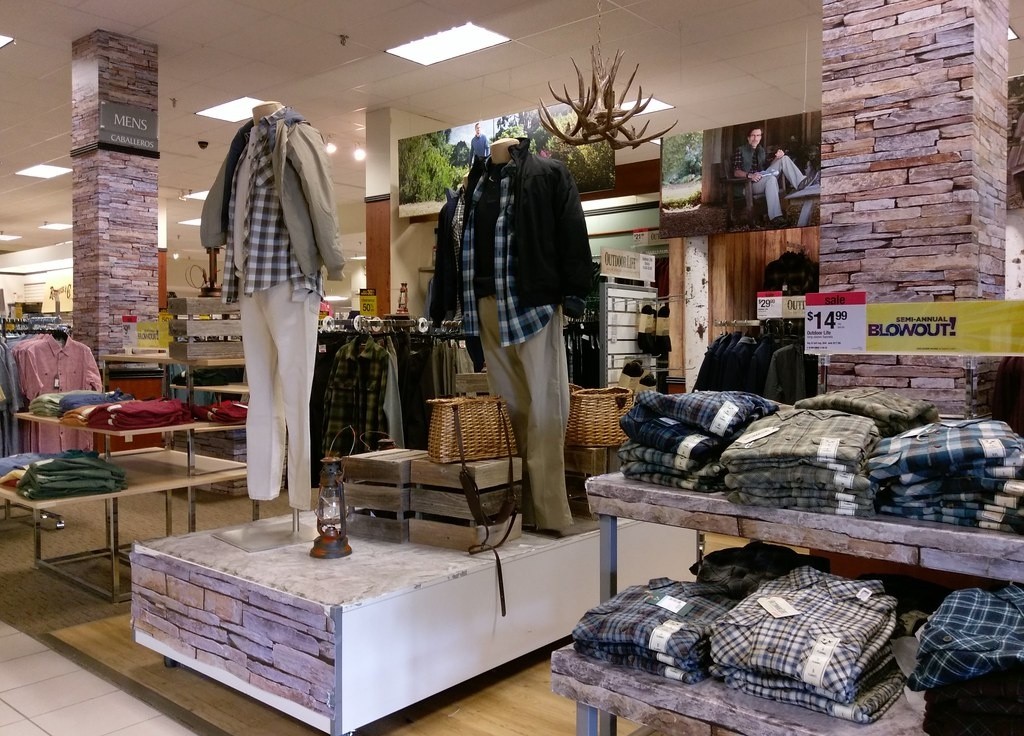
[798, 170, 819, 191]
[766, 216, 794, 229]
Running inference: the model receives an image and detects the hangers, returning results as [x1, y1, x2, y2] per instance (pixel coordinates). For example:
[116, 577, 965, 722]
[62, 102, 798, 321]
[716, 320, 820, 351]
[318, 312, 473, 347]
[0, 316, 72, 339]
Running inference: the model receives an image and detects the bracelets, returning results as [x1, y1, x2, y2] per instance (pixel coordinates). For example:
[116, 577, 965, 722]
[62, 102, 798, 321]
[746, 171, 752, 178]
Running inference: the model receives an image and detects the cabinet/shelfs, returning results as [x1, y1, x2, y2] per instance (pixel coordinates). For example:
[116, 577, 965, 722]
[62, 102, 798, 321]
[0, 347, 248, 604]
[552, 472, 1024, 736]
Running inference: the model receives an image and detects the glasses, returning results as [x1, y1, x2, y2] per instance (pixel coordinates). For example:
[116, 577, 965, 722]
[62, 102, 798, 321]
[749, 134, 762, 137]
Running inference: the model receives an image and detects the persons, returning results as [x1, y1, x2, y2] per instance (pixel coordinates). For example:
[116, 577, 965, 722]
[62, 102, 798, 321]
[469, 123, 489, 168]
[429, 171, 485, 374]
[201, 102, 344, 509]
[732, 126, 818, 229]
[459, 139, 593, 530]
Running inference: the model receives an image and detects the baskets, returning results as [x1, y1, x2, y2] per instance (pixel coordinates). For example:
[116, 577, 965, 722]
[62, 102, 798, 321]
[565, 386, 634, 446]
[426, 395, 518, 464]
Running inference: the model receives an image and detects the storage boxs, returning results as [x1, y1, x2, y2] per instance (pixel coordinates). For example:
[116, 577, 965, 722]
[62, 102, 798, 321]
[166, 297, 244, 361]
[562, 444, 622, 521]
[341, 448, 429, 544]
[408, 454, 524, 552]
